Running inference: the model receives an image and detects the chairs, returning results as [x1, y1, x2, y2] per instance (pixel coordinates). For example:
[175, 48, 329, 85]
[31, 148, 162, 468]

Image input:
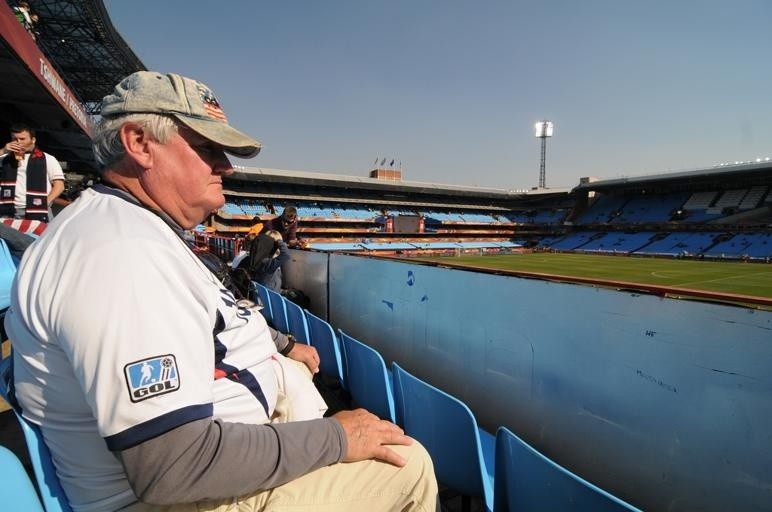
[336, 326, 396, 427]
[390, 359, 498, 510]
[0, 232, 70, 512]
[266, 287, 289, 334]
[281, 296, 310, 350]
[489, 423, 641, 510]
[254, 282, 274, 320]
[302, 307, 343, 381]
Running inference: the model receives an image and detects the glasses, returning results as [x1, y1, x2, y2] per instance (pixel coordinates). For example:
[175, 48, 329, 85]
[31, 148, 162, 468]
[229, 281, 265, 312]
[282, 216, 294, 224]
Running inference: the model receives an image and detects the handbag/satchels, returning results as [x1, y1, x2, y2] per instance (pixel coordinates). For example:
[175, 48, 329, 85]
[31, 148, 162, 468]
[286, 290, 309, 309]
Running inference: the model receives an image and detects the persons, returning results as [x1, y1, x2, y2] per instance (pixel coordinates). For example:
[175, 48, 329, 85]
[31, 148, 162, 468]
[13, 2, 32, 30]
[181, 206, 313, 309]
[51, 173, 101, 219]
[2, 121, 67, 241]
[5, 68, 443, 512]
[24, 14, 41, 43]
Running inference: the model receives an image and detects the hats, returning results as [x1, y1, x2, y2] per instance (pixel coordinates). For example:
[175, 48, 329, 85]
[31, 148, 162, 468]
[100, 70, 261, 160]
